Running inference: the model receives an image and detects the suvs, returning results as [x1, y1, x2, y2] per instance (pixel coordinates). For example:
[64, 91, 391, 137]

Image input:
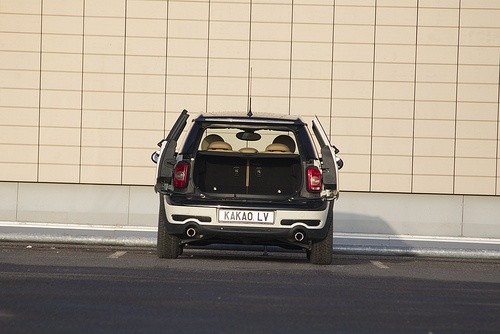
[151, 105, 343, 265]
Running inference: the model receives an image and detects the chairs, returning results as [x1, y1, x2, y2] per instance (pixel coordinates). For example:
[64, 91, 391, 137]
[201, 134, 224, 150]
[272, 134, 296, 153]
[265, 144, 290, 154]
[207, 142, 236, 152]
[239, 148, 257, 153]
